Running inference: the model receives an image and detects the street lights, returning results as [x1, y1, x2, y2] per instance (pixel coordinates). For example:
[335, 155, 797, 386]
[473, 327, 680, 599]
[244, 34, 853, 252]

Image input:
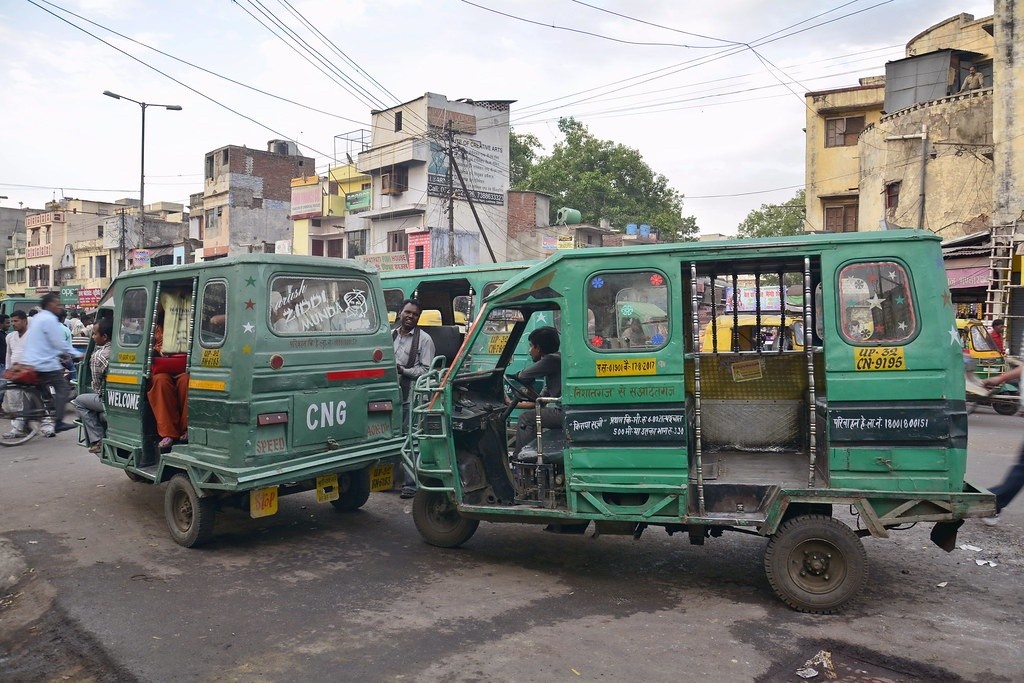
[102, 90, 183, 247]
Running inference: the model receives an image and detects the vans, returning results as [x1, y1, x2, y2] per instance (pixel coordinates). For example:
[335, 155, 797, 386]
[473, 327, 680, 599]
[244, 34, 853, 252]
[399, 227, 998, 617]
[0, 297, 43, 332]
[69, 253, 408, 549]
[380, 259, 664, 447]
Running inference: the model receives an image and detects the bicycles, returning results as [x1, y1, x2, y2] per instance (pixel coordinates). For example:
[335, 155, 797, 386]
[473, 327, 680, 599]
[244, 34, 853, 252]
[0, 358, 84, 447]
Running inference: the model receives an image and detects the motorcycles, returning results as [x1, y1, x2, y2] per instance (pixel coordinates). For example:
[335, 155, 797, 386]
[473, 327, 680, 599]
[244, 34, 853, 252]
[701, 313, 805, 352]
[861, 317, 1020, 416]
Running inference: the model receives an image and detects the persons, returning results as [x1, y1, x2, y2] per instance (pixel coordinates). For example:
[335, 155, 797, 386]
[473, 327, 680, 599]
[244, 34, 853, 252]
[74, 319, 112, 453]
[391, 299, 435, 497]
[32, 308, 93, 389]
[980, 366, 1024, 525]
[622, 318, 651, 345]
[0, 310, 56, 439]
[509, 326, 562, 462]
[18, 295, 83, 436]
[270, 279, 330, 331]
[147, 325, 190, 447]
[990, 319, 1004, 353]
[209, 305, 225, 336]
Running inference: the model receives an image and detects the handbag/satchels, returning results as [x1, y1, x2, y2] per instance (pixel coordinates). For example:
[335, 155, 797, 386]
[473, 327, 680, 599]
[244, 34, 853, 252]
[151, 353, 187, 375]
[2, 362, 39, 386]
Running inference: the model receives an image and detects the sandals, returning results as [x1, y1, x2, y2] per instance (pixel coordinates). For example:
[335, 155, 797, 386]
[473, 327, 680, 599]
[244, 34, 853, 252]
[158, 437, 174, 447]
[179, 432, 188, 441]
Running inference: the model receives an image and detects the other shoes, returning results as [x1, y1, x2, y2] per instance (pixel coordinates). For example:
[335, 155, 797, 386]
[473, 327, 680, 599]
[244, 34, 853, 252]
[55, 423, 78, 433]
[42, 430, 56, 437]
[88, 441, 101, 453]
[981, 517, 999, 526]
[2, 427, 26, 439]
[400, 488, 417, 498]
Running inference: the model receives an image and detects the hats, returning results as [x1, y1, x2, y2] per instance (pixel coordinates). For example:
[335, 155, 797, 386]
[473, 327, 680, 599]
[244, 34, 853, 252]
[271, 291, 283, 309]
